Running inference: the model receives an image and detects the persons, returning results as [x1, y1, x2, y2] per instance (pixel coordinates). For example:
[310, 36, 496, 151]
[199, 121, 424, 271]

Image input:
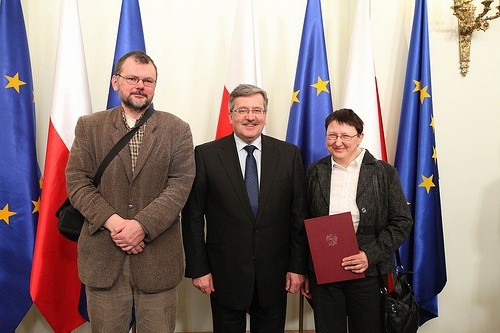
[300, 108, 413, 333]
[64, 51, 196, 333]
[180, 84, 308, 333]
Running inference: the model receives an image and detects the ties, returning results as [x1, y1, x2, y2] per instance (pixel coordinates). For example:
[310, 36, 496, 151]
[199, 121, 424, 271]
[241, 145, 260, 222]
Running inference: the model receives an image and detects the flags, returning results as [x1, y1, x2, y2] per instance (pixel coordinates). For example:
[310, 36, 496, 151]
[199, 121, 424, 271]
[0, 0, 42, 333]
[30, 1, 92, 333]
[285, 0, 336, 169]
[392, 0, 448, 333]
[341, 1, 395, 292]
[214, 0, 266, 140]
[105, 0, 156, 108]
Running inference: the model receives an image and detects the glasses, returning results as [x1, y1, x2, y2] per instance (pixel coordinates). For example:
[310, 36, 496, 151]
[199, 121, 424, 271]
[232, 108, 264, 115]
[118, 74, 158, 88]
[325, 133, 358, 141]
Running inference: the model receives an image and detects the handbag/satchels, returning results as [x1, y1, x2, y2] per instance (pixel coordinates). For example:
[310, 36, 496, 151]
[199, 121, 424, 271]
[54, 197, 85, 243]
[380, 264, 420, 333]
